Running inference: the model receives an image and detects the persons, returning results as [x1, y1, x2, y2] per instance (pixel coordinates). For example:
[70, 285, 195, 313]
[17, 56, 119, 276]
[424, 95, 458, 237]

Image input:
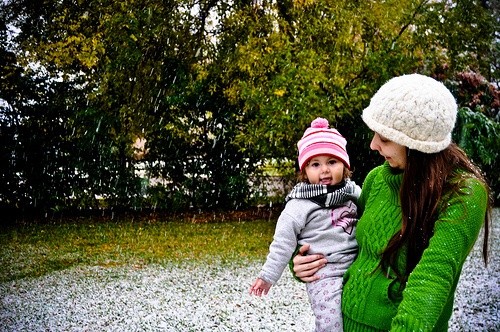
[249, 117, 363, 332]
[288, 72, 491, 332]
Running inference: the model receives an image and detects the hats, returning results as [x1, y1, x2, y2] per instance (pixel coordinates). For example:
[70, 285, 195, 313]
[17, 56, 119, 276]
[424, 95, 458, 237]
[296, 117, 350, 171]
[361, 74, 458, 153]
